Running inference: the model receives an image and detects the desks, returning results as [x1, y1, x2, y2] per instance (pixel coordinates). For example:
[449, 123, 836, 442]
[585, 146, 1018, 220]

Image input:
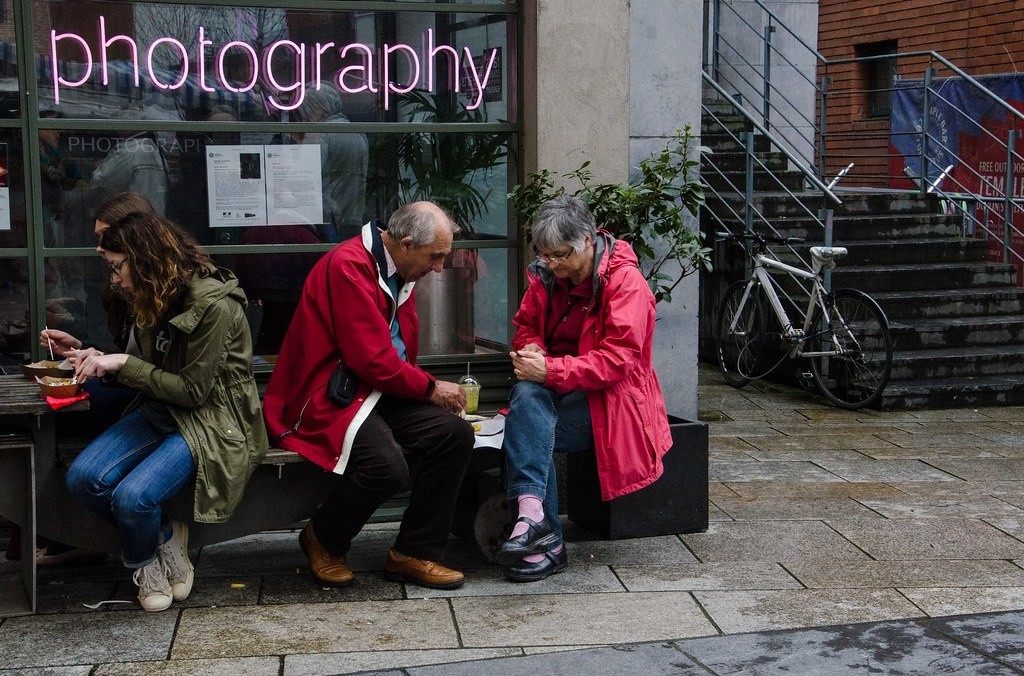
[0, 363, 124, 567]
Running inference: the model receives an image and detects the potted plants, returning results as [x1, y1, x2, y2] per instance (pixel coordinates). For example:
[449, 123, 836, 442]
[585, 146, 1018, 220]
[507, 125, 710, 537]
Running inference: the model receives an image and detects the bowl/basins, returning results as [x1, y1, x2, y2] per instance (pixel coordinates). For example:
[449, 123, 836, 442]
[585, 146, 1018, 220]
[22, 359, 86, 395]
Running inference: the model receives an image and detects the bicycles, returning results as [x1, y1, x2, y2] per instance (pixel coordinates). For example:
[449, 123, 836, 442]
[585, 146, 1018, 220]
[715, 231, 895, 410]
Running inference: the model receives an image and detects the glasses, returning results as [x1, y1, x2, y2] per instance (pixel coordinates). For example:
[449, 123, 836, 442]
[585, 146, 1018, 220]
[111, 256, 129, 276]
[536, 247, 574, 263]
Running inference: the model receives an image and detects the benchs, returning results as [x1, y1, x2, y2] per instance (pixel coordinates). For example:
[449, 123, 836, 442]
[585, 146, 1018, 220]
[177, 350, 513, 550]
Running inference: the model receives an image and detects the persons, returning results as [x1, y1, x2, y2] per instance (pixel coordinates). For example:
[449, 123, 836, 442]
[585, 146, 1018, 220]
[495, 195, 675, 583]
[261, 201, 476, 589]
[39, 192, 269, 612]
[9, 78, 372, 366]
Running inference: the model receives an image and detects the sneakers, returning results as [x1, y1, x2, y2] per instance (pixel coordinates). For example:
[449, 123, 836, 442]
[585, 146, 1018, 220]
[158, 517, 195, 601]
[132, 558, 174, 612]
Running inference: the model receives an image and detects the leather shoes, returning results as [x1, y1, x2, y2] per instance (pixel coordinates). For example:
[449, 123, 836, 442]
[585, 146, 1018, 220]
[506, 543, 569, 582]
[299, 519, 354, 587]
[385, 547, 465, 591]
[496, 517, 559, 565]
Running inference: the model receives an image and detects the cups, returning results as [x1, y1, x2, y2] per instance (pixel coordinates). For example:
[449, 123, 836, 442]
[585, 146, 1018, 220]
[459, 375, 481, 414]
